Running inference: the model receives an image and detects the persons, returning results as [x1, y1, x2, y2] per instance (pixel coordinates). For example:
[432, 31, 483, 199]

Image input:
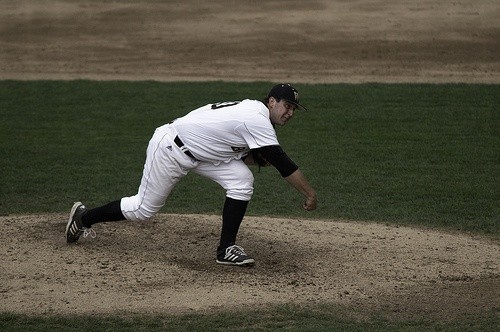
[64, 83, 317, 265]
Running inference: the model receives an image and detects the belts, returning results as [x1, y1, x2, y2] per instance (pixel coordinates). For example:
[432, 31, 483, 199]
[174, 136, 200, 161]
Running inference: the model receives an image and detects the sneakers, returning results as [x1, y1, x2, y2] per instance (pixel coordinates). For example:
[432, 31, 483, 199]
[65, 201, 96, 246]
[216, 245, 255, 267]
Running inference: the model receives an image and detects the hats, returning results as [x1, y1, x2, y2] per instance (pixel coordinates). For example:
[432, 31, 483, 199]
[268, 83, 308, 112]
